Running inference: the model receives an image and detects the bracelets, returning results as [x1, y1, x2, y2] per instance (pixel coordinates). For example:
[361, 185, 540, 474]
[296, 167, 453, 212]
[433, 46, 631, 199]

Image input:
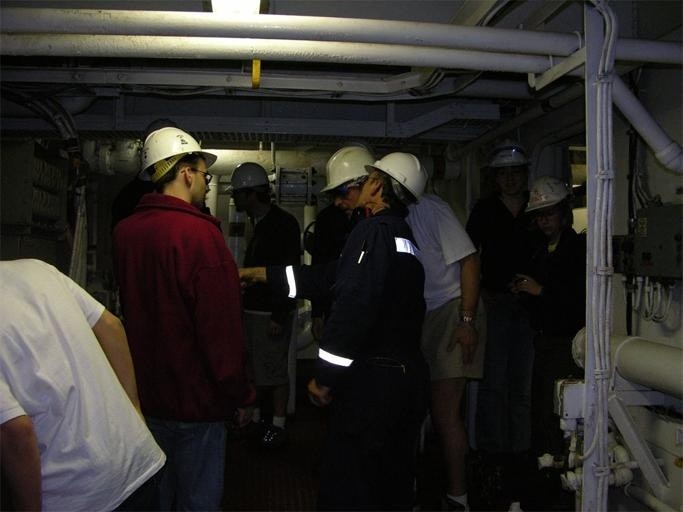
[458, 311, 476, 329]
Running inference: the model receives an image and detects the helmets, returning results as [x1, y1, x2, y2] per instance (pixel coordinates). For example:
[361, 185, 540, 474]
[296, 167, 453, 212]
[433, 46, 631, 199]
[225, 162, 269, 192]
[320, 146, 428, 203]
[141, 127, 216, 174]
[482, 148, 574, 211]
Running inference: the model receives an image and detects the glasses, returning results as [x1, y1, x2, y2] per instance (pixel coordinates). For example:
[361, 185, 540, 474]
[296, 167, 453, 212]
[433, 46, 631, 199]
[181, 168, 212, 184]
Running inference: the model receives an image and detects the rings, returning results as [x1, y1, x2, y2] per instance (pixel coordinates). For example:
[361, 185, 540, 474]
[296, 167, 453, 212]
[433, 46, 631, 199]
[523, 278, 528, 283]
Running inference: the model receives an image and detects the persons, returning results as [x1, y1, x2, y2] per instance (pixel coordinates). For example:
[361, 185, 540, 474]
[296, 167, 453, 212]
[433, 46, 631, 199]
[504, 173, 595, 382]
[0, 258, 171, 512]
[236, 150, 431, 512]
[112, 127, 244, 512]
[319, 144, 487, 510]
[225, 159, 302, 449]
[465, 141, 533, 332]
